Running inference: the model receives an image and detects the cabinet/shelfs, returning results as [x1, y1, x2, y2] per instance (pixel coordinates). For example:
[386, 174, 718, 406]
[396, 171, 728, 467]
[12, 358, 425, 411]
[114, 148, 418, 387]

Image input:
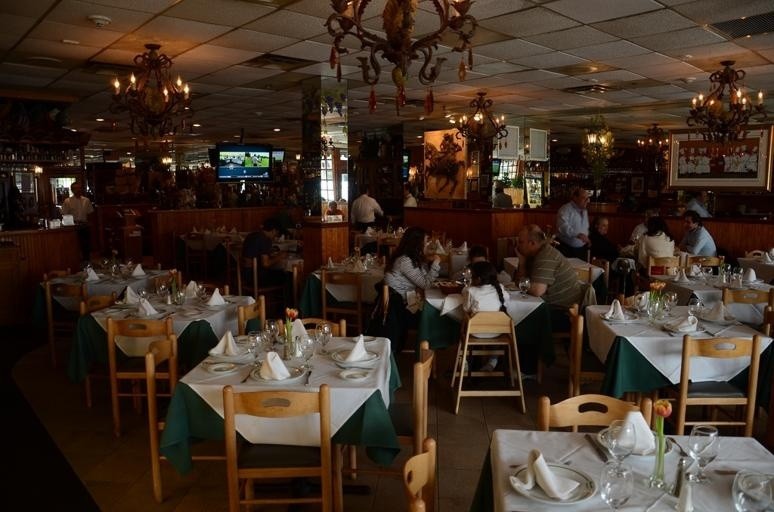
[0, 90, 91, 225]
[404, 207, 559, 263]
[591, 213, 774, 261]
[1, 219, 80, 328]
[91, 163, 151, 256]
[152, 204, 303, 262]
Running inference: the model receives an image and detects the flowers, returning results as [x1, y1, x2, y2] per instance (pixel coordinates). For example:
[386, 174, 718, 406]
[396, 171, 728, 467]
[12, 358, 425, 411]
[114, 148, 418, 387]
[285, 306, 299, 344]
[719, 256, 725, 276]
[110, 249, 119, 268]
[657, 282, 666, 305]
[649, 281, 658, 306]
[171, 268, 179, 295]
[653, 398, 673, 479]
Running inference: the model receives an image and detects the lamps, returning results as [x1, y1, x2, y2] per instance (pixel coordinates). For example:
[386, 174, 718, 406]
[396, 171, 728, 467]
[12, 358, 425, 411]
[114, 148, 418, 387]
[634, 124, 672, 167]
[687, 62, 763, 158]
[582, 117, 617, 190]
[325, 1, 477, 118]
[105, 43, 191, 137]
[444, 91, 509, 159]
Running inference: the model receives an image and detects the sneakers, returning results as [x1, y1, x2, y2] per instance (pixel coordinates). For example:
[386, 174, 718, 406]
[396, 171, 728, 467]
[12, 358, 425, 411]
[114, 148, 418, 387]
[479, 363, 494, 372]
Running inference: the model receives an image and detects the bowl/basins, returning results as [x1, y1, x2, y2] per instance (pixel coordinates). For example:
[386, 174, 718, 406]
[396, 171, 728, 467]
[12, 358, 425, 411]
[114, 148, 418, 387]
[438, 280, 466, 295]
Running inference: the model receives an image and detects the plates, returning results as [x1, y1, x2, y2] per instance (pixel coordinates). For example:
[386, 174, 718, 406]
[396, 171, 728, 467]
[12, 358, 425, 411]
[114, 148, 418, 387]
[322, 265, 339, 271]
[352, 336, 377, 344]
[129, 308, 167, 317]
[742, 278, 764, 284]
[625, 307, 649, 317]
[208, 305, 225, 311]
[738, 472, 774, 501]
[207, 363, 237, 374]
[236, 335, 248, 343]
[668, 277, 697, 285]
[596, 425, 674, 456]
[699, 309, 737, 325]
[182, 310, 204, 317]
[510, 462, 598, 505]
[336, 369, 371, 381]
[664, 319, 708, 336]
[80, 276, 105, 284]
[600, 312, 639, 322]
[330, 348, 380, 364]
[129, 275, 149, 280]
[344, 269, 368, 274]
[753, 258, 774, 264]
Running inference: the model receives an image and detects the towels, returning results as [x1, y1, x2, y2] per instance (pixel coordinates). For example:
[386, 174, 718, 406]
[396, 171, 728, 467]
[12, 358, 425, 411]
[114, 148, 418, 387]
[704, 300, 731, 321]
[671, 267, 689, 283]
[259, 352, 291, 380]
[671, 315, 699, 332]
[612, 410, 655, 456]
[85, 268, 99, 281]
[600, 299, 624, 320]
[439, 293, 464, 316]
[130, 298, 158, 316]
[509, 448, 580, 501]
[208, 330, 239, 356]
[455, 241, 467, 251]
[205, 287, 228, 306]
[115, 286, 141, 305]
[185, 280, 197, 298]
[352, 259, 365, 272]
[291, 318, 308, 343]
[743, 268, 757, 281]
[131, 264, 145, 276]
[334, 333, 376, 363]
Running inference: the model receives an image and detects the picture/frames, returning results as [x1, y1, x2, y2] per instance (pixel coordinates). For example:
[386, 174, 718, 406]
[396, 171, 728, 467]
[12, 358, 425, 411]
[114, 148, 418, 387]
[422, 129, 468, 200]
[666, 126, 773, 192]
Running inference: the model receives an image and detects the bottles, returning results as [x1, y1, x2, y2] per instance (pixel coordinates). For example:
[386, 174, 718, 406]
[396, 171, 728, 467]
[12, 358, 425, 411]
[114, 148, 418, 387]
[292, 336, 302, 358]
[672, 458, 687, 497]
[166, 291, 172, 305]
[282, 337, 291, 360]
[674, 473, 694, 512]
[176, 290, 182, 305]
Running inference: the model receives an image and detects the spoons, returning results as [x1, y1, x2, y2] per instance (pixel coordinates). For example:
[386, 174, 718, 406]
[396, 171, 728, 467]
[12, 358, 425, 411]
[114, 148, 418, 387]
[669, 437, 688, 457]
[600, 459, 634, 512]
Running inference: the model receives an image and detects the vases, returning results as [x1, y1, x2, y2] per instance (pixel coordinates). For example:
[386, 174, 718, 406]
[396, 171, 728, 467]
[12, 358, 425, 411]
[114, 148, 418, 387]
[649, 435, 668, 491]
[719, 271, 724, 283]
[648, 300, 656, 326]
[285, 333, 294, 355]
[658, 301, 664, 320]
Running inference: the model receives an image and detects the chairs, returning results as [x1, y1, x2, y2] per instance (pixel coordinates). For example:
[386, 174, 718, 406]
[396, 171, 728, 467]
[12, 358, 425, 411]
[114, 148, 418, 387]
[745, 251, 763, 255]
[45, 268, 71, 281]
[294, 264, 308, 307]
[568, 303, 636, 434]
[383, 282, 447, 379]
[236, 255, 292, 301]
[322, 269, 375, 335]
[223, 238, 243, 262]
[648, 255, 684, 278]
[345, 340, 434, 480]
[237, 296, 265, 334]
[763, 307, 773, 336]
[45, 282, 87, 364]
[302, 316, 346, 337]
[537, 267, 593, 383]
[224, 385, 345, 511]
[360, 240, 388, 260]
[540, 394, 653, 434]
[451, 311, 527, 415]
[80, 291, 142, 410]
[590, 257, 610, 304]
[153, 270, 182, 294]
[665, 334, 760, 435]
[199, 282, 227, 295]
[403, 438, 437, 512]
[107, 317, 178, 439]
[723, 288, 774, 321]
[686, 254, 724, 276]
[144, 334, 256, 511]
[739, 253, 773, 284]
[186, 232, 205, 268]
[144, 262, 161, 269]
[650, 279, 696, 306]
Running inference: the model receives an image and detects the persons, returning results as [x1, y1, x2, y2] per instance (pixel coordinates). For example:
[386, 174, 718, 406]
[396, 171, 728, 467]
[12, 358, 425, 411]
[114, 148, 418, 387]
[459, 261, 510, 373]
[679, 146, 758, 174]
[590, 216, 624, 264]
[63, 183, 94, 262]
[679, 210, 719, 275]
[271, 203, 304, 231]
[351, 183, 384, 233]
[379, 225, 441, 331]
[241, 220, 290, 288]
[617, 216, 675, 275]
[251, 154, 261, 166]
[494, 186, 512, 206]
[515, 224, 582, 381]
[452, 246, 511, 283]
[556, 187, 589, 262]
[686, 189, 712, 217]
[627, 208, 659, 244]
[588, 217, 618, 261]
[403, 181, 418, 207]
[325, 201, 345, 220]
[437, 133, 462, 161]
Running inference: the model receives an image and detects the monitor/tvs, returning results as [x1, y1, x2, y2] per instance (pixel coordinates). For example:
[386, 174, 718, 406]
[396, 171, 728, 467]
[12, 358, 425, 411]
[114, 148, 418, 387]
[214, 143, 272, 182]
[491, 158, 501, 176]
[403, 154, 410, 183]
[272, 149, 286, 162]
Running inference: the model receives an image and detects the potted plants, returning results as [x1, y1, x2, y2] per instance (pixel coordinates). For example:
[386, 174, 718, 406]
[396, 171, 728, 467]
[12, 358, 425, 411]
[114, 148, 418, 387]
[496, 172, 511, 188]
[512, 176, 524, 188]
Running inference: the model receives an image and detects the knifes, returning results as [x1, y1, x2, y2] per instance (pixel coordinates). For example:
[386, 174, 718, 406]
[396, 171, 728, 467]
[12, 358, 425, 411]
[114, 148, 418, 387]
[584, 434, 608, 463]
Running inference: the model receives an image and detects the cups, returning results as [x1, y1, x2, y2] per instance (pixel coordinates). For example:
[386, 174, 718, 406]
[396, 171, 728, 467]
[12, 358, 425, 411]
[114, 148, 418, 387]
[119, 271, 130, 285]
[634, 293, 647, 317]
[687, 425, 719, 484]
[702, 267, 713, 281]
[733, 267, 744, 280]
[315, 323, 332, 355]
[691, 263, 702, 281]
[604, 420, 636, 474]
[646, 300, 661, 327]
[732, 468, 772, 512]
[688, 298, 702, 317]
[248, 331, 265, 367]
[297, 334, 315, 371]
[721, 264, 731, 283]
[249, 366, 307, 384]
[518, 277, 530, 299]
[664, 291, 678, 316]
[263, 319, 280, 352]
